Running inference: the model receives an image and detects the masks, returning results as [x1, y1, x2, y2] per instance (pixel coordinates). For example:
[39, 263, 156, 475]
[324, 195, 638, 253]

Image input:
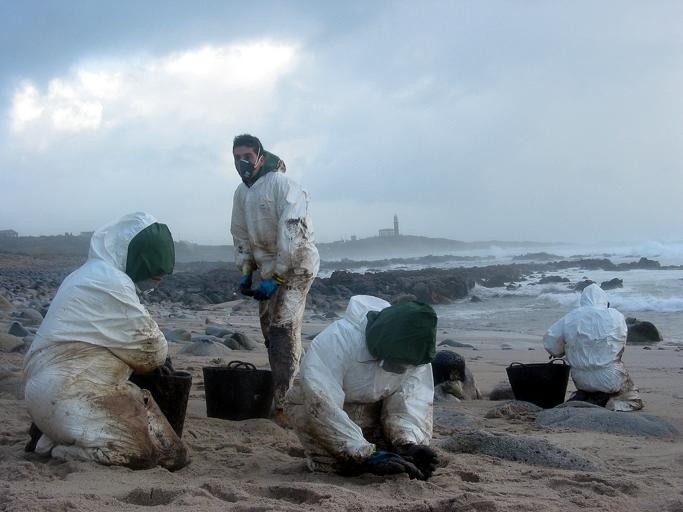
[233, 159, 253, 180]
[131, 278, 159, 298]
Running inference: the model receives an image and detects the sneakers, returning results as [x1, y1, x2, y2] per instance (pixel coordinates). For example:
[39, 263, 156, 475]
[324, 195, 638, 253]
[23, 423, 42, 453]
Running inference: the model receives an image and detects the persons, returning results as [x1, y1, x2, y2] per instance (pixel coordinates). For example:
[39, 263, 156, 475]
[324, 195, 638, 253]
[539, 283, 645, 414]
[281, 291, 443, 482]
[227, 132, 321, 432]
[17, 210, 192, 472]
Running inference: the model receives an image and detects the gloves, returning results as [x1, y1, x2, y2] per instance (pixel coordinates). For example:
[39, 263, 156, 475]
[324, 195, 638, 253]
[238, 271, 254, 298]
[369, 448, 423, 479]
[154, 354, 175, 377]
[251, 273, 284, 303]
[395, 442, 440, 482]
